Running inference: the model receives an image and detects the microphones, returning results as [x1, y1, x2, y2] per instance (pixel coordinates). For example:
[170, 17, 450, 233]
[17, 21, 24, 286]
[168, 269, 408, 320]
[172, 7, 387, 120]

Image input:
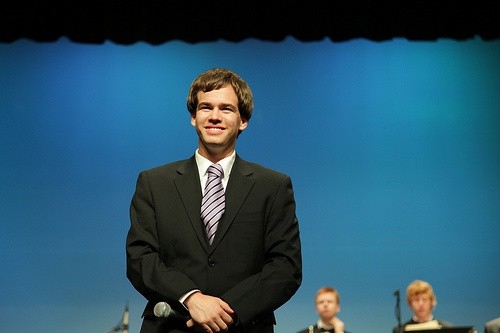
[394, 290, 402, 333]
[154, 302, 192, 322]
[122, 306, 129, 333]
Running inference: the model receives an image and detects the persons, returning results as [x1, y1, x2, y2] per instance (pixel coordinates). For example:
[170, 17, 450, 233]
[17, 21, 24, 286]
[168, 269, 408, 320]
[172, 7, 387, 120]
[296, 287, 352, 333]
[392, 279, 477, 333]
[125, 68, 302, 333]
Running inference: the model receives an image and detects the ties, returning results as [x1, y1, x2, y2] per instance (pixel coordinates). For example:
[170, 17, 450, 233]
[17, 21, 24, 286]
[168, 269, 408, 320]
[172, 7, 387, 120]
[199, 164, 227, 246]
